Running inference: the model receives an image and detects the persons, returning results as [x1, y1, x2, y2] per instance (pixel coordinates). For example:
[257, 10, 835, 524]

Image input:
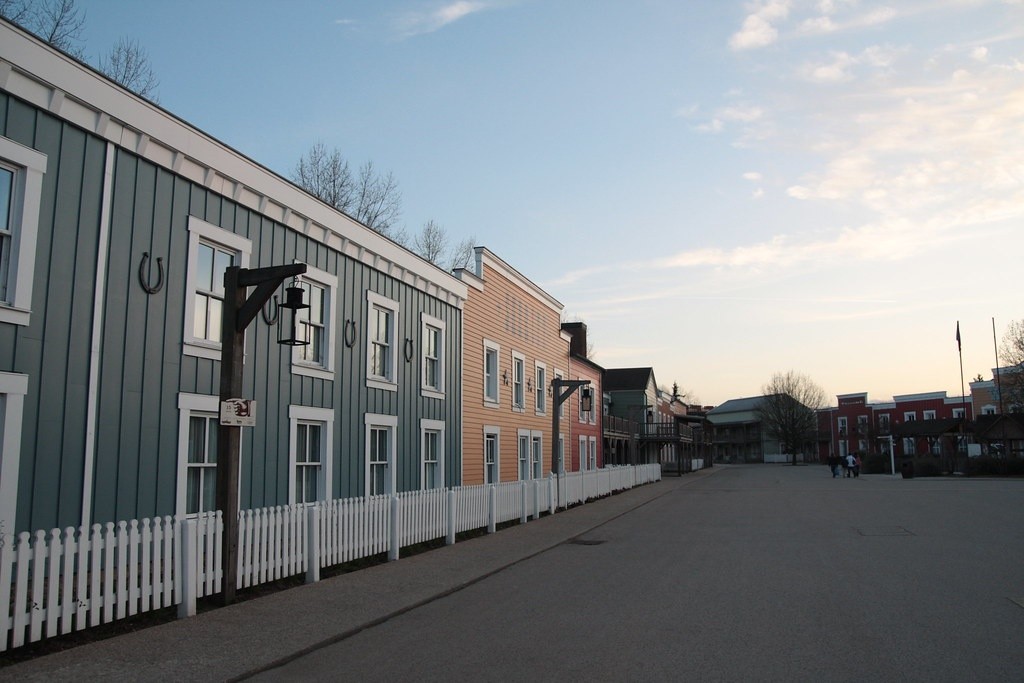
[828, 453, 838, 478]
[843, 452, 861, 478]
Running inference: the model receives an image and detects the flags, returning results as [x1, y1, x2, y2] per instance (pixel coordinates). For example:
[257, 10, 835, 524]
[956, 325, 961, 350]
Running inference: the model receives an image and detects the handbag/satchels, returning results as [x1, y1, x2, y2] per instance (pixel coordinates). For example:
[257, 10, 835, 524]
[852, 459, 855, 465]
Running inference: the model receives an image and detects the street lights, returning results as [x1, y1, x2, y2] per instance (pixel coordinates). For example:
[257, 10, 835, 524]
[214, 263, 309, 609]
[876, 435, 895, 475]
[550, 377, 592, 474]
